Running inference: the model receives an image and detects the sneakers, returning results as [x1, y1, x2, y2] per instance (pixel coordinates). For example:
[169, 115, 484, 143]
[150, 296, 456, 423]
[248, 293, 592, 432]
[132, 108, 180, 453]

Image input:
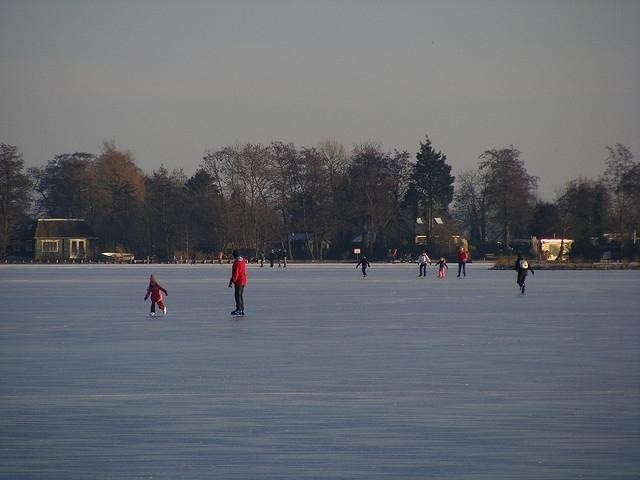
[149, 312, 155, 316]
[163, 307, 167, 315]
[231, 311, 245, 314]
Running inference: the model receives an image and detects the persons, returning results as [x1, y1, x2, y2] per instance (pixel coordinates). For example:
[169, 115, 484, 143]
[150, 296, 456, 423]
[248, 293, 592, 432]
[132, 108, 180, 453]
[355, 255, 371, 277]
[226, 247, 248, 316]
[143, 273, 168, 317]
[283, 247, 287, 267]
[391, 248, 398, 263]
[514, 252, 535, 294]
[269, 248, 274, 267]
[435, 255, 449, 279]
[456, 246, 469, 277]
[416, 249, 432, 277]
[278, 249, 281, 267]
[258, 251, 265, 266]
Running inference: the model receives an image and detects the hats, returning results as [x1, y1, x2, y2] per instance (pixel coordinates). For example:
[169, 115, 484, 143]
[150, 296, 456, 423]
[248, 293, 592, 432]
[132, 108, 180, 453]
[150, 274, 157, 284]
